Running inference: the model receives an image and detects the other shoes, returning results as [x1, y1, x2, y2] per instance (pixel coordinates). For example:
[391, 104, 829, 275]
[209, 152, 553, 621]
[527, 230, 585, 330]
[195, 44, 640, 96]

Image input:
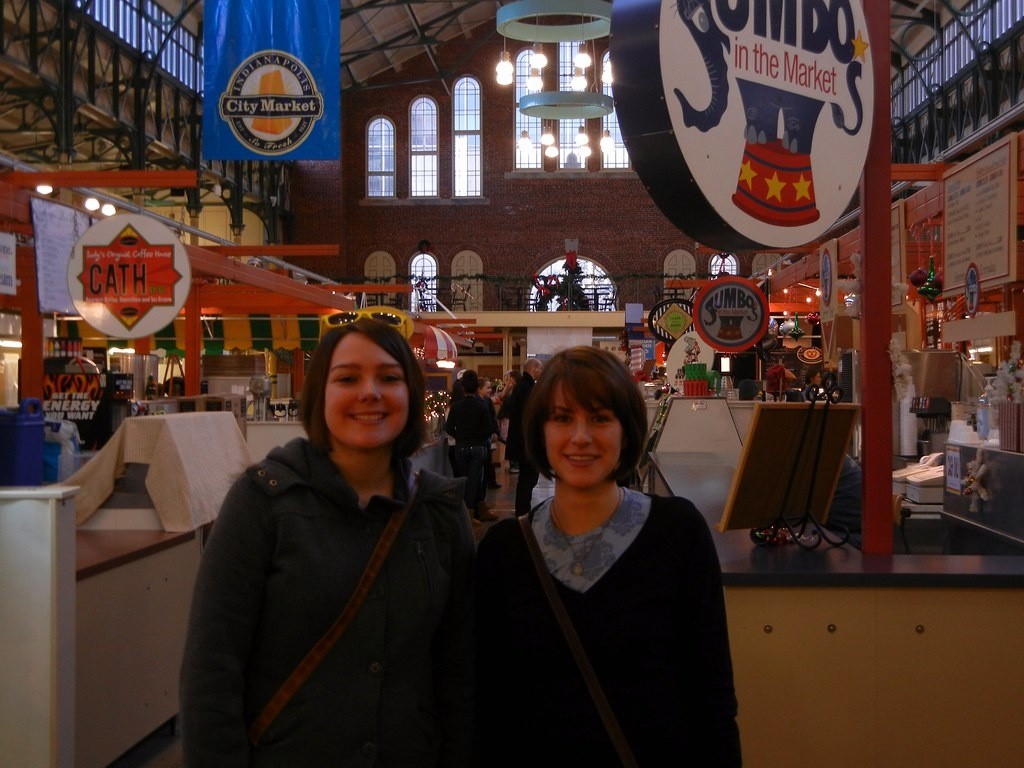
[510, 467, 520, 473]
[488, 483, 502, 489]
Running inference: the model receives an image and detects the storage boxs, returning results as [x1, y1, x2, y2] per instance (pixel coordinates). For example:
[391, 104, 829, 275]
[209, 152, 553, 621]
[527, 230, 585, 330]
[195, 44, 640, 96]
[0, 397, 81, 486]
[737, 379, 763, 401]
[999, 402, 1020, 452]
[176, 391, 228, 412]
[685, 363, 707, 380]
[943, 442, 1024, 541]
[720, 388, 739, 400]
[693, 401, 707, 410]
[201, 395, 247, 443]
[1018, 402, 1024, 454]
[893, 481, 907, 498]
[683, 380, 709, 397]
[905, 484, 943, 505]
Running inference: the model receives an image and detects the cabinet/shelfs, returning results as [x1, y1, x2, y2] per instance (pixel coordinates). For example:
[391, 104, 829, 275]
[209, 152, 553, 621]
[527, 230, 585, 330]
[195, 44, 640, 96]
[724, 586, 1024, 768]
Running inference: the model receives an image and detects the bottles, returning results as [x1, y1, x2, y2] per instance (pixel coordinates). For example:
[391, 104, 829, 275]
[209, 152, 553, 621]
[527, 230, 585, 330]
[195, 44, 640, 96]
[146, 375, 159, 401]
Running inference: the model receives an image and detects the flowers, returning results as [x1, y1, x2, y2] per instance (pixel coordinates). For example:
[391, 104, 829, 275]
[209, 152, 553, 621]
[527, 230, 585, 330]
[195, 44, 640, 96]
[490, 378, 505, 390]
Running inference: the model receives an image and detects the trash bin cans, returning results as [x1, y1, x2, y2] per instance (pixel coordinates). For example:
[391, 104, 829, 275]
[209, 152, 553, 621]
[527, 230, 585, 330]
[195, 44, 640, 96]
[44, 420, 80, 482]
[0, 397, 45, 486]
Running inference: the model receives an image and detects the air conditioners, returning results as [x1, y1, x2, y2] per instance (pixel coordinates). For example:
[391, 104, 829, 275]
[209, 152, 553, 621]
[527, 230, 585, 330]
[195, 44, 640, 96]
[837, 349, 862, 459]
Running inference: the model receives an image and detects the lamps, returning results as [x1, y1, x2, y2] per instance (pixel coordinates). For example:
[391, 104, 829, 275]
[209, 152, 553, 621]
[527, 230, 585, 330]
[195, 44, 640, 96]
[515, 90, 615, 160]
[494, 0, 614, 90]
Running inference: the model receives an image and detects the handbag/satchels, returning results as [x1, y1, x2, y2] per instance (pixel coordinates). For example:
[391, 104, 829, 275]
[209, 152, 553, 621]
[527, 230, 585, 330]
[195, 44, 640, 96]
[462, 443, 483, 459]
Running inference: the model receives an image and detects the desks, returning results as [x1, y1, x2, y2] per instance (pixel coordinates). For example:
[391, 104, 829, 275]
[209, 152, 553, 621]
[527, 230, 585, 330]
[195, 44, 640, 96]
[938, 512, 1024, 551]
[245, 420, 309, 465]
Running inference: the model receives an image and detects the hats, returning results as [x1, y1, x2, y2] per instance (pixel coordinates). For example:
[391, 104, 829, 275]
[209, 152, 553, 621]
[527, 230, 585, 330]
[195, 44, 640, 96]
[766, 365, 785, 393]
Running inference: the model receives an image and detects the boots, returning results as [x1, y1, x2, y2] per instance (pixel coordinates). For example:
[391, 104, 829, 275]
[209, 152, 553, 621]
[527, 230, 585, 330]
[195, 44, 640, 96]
[475, 501, 499, 521]
[467, 509, 482, 526]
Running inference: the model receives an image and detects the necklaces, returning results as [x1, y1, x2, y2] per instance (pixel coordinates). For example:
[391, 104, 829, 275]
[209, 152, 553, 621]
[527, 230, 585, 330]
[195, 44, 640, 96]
[548, 487, 621, 577]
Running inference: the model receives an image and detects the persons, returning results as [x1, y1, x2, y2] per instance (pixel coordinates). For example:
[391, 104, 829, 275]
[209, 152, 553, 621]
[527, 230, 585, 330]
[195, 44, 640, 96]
[446, 359, 543, 517]
[181, 319, 488, 768]
[481, 345, 744, 767]
[802, 368, 821, 400]
[829, 453, 862, 543]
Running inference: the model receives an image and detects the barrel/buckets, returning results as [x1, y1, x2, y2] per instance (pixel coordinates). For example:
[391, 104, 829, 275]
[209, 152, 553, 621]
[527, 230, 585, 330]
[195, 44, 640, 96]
[0, 396, 46, 487]
